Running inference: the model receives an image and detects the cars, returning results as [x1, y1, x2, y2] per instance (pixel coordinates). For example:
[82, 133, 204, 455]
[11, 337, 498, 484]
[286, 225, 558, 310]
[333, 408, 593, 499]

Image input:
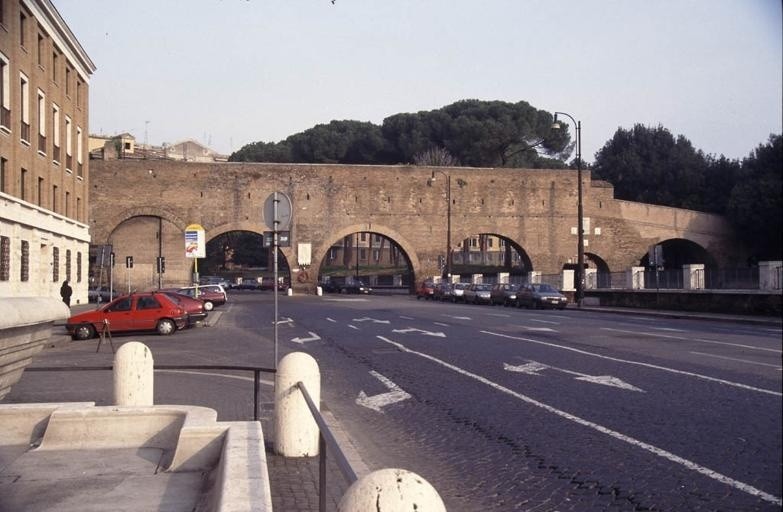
[418, 281, 568, 309]
[66, 275, 290, 340]
[319, 280, 372, 294]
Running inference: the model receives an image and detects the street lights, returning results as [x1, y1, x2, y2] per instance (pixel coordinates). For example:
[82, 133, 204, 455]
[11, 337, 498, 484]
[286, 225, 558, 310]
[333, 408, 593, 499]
[430, 170, 452, 282]
[551, 111, 583, 302]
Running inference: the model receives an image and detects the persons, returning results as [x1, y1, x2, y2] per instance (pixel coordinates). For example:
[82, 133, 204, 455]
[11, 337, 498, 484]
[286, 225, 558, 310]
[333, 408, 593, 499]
[59, 278, 72, 308]
